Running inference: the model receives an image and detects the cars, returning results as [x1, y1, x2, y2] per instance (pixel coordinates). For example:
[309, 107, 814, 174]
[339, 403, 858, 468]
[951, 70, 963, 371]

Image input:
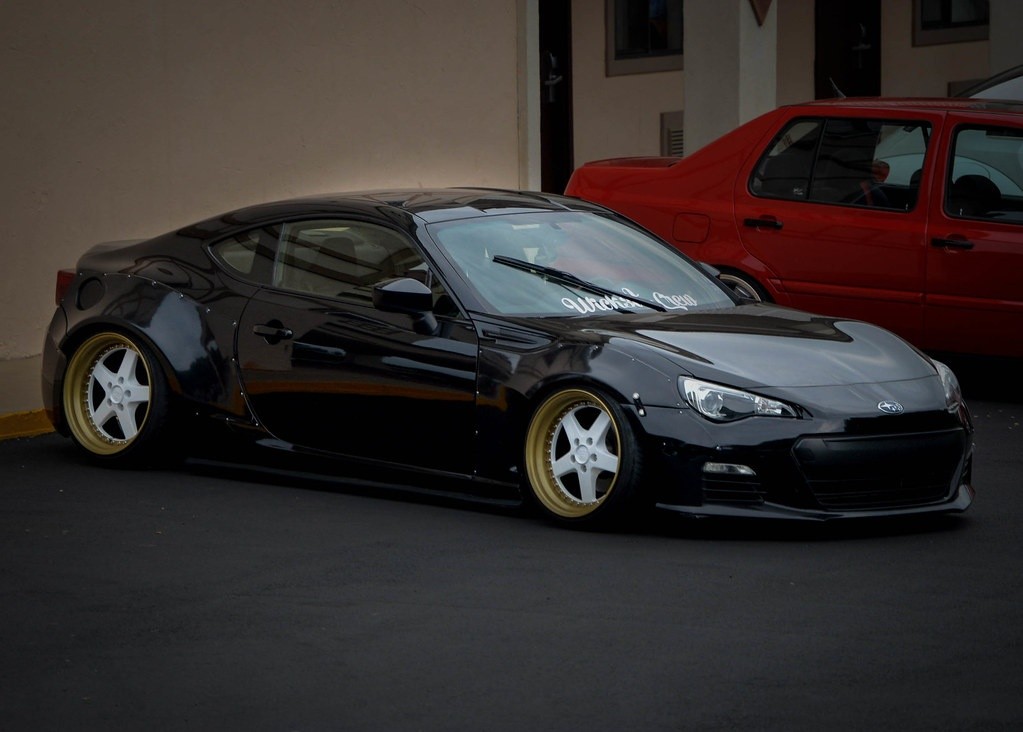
[533, 98, 1023, 365]
[874, 66, 1023, 221]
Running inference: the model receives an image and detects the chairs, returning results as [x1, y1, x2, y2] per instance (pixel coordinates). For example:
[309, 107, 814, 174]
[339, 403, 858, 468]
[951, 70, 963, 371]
[910, 168, 963, 213]
[955, 175, 1002, 216]
[300, 237, 358, 290]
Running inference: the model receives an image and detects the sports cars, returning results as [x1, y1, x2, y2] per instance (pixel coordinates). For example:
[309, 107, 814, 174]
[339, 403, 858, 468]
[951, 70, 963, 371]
[42, 187, 978, 532]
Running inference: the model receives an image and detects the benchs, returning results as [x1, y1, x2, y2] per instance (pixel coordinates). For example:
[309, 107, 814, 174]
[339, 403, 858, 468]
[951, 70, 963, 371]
[790, 166, 890, 209]
